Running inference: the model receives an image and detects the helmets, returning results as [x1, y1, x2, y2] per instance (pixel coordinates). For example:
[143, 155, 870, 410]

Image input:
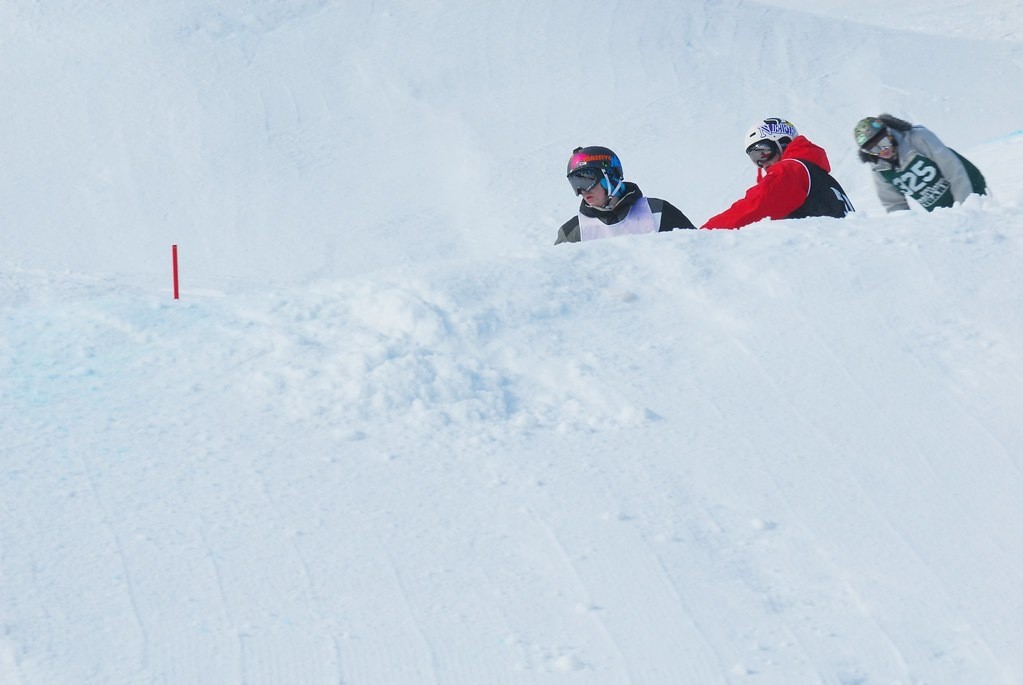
[567, 146, 626, 197]
[855, 118, 886, 146]
[745, 117, 798, 155]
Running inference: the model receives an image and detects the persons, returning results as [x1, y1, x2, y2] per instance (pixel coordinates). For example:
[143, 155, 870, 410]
[554, 146, 697, 246]
[856, 114, 987, 213]
[700, 118, 855, 230]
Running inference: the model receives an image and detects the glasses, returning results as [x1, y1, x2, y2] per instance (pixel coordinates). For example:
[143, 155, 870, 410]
[748, 141, 776, 168]
[567, 168, 601, 197]
[860, 128, 893, 156]
